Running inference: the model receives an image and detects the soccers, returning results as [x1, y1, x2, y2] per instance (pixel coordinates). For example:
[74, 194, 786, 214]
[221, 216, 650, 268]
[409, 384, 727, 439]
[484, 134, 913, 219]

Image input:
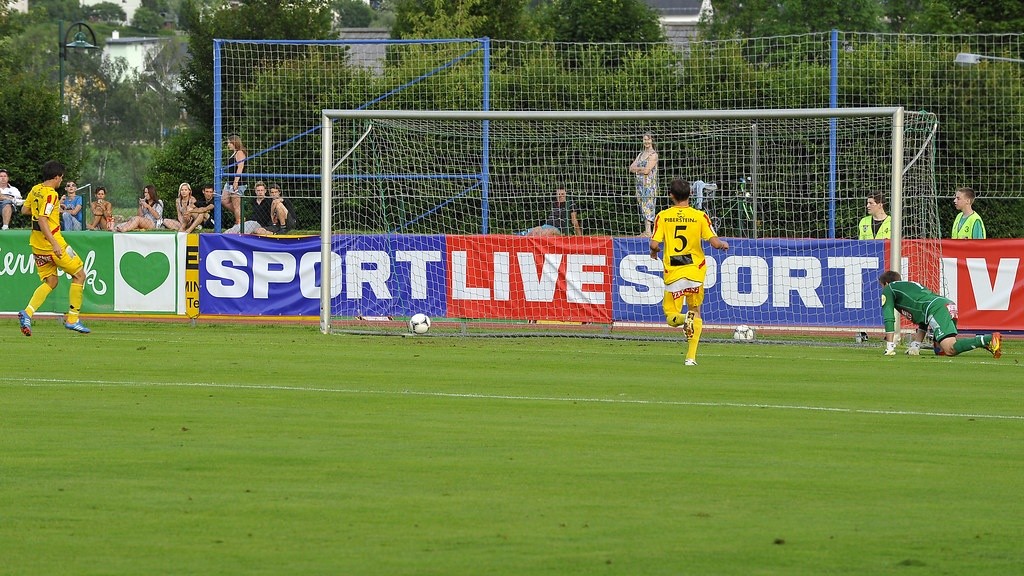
[409, 313, 431, 335]
[735, 324, 754, 340]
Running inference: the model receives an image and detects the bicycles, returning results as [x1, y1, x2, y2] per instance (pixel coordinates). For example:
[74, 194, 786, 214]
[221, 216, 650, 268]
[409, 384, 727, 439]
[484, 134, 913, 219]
[692, 177, 776, 238]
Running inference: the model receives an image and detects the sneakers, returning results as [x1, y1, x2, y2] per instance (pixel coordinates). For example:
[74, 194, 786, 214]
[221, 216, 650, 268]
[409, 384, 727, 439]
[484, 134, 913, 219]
[987, 332, 1003, 358]
[683, 311, 696, 340]
[17, 310, 31, 336]
[684, 360, 697, 366]
[65, 320, 93, 334]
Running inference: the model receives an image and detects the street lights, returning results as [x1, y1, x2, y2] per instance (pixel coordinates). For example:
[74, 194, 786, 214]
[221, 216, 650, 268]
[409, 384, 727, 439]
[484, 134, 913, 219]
[58, 22, 102, 104]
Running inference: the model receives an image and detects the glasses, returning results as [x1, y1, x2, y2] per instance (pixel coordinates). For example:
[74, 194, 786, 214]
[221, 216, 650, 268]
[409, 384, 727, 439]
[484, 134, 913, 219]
[65, 184, 76, 188]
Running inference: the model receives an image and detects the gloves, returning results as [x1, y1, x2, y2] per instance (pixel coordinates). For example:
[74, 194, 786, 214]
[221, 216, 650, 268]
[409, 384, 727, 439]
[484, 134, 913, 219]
[904, 341, 921, 356]
[884, 341, 896, 356]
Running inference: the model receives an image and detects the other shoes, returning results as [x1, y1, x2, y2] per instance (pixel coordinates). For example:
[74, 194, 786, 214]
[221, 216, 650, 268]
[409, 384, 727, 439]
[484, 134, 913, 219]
[2, 224, 9, 231]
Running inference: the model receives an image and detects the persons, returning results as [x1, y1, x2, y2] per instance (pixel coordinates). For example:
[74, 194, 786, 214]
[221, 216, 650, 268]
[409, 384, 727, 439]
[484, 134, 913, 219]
[18, 160, 91, 336]
[60, 180, 82, 232]
[0, 169, 22, 230]
[629, 133, 659, 236]
[651, 179, 729, 365]
[878, 271, 1002, 359]
[86, 187, 112, 231]
[951, 188, 987, 336]
[511, 187, 581, 236]
[857, 194, 892, 342]
[222, 135, 248, 225]
[117, 182, 295, 235]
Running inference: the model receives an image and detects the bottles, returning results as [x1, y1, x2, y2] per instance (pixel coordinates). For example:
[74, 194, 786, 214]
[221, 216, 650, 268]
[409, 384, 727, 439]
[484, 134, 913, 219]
[143, 197, 146, 202]
